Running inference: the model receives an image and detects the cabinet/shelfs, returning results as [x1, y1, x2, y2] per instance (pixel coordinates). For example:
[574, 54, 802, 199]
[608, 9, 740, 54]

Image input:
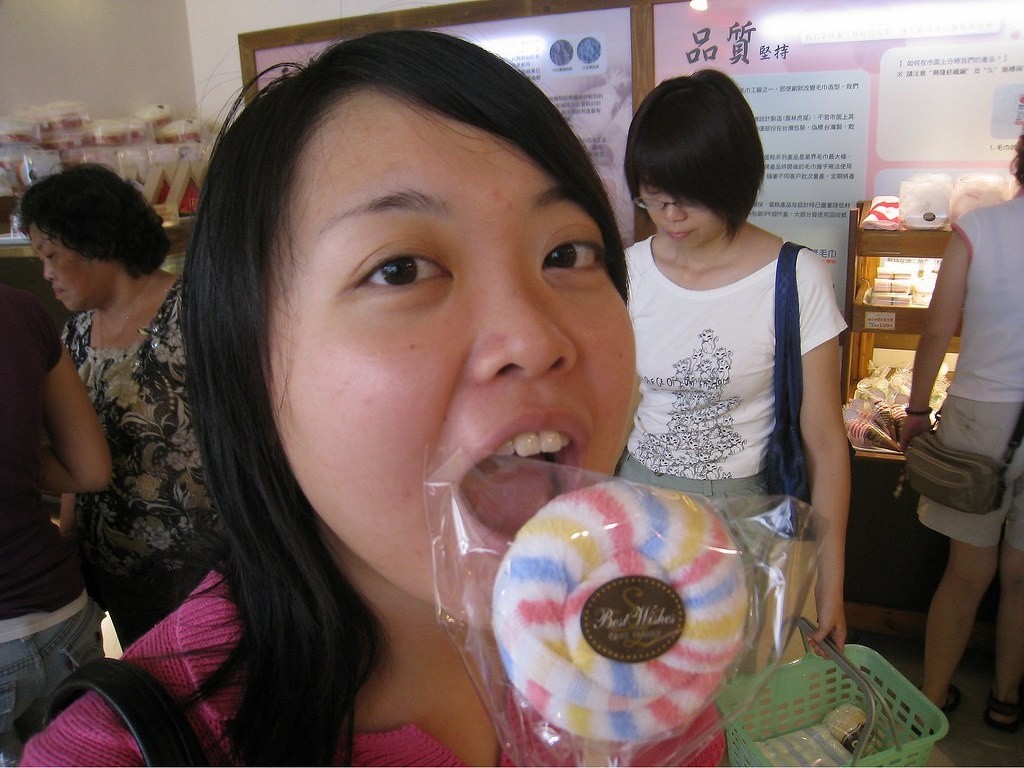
[840, 200, 1002, 654]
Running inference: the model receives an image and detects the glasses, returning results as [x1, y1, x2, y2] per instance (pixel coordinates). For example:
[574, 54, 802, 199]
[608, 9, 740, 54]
[633, 188, 711, 211]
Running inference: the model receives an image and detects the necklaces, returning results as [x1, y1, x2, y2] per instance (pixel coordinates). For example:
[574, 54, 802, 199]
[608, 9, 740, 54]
[99, 310, 130, 352]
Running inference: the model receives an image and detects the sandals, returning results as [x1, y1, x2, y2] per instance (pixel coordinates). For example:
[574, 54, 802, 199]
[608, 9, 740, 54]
[983, 680, 1024, 734]
[906, 682, 960, 736]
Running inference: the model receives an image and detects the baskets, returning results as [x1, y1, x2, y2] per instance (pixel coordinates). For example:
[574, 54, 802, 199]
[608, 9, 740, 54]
[712, 616, 948, 767]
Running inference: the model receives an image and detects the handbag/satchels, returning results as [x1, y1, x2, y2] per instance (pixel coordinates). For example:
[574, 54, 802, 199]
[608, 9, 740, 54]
[766, 242, 856, 506]
[903, 431, 1007, 515]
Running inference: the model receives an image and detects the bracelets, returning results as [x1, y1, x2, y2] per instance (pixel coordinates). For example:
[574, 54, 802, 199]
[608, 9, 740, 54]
[905, 408, 933, 416]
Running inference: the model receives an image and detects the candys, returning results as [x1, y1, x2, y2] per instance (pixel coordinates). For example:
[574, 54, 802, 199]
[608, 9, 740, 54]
[491, 480, 751, 767]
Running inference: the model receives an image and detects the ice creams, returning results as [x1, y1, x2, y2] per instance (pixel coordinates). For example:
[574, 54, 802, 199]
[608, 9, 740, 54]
[844, 398, 911, 453]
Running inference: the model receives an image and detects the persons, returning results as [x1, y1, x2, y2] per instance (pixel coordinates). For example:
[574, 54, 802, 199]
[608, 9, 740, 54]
[0, 163, 218, 767]
[900, 134, 1024, 727]
[18, 28, 726, 768]
[622, 68, 847, 661]
